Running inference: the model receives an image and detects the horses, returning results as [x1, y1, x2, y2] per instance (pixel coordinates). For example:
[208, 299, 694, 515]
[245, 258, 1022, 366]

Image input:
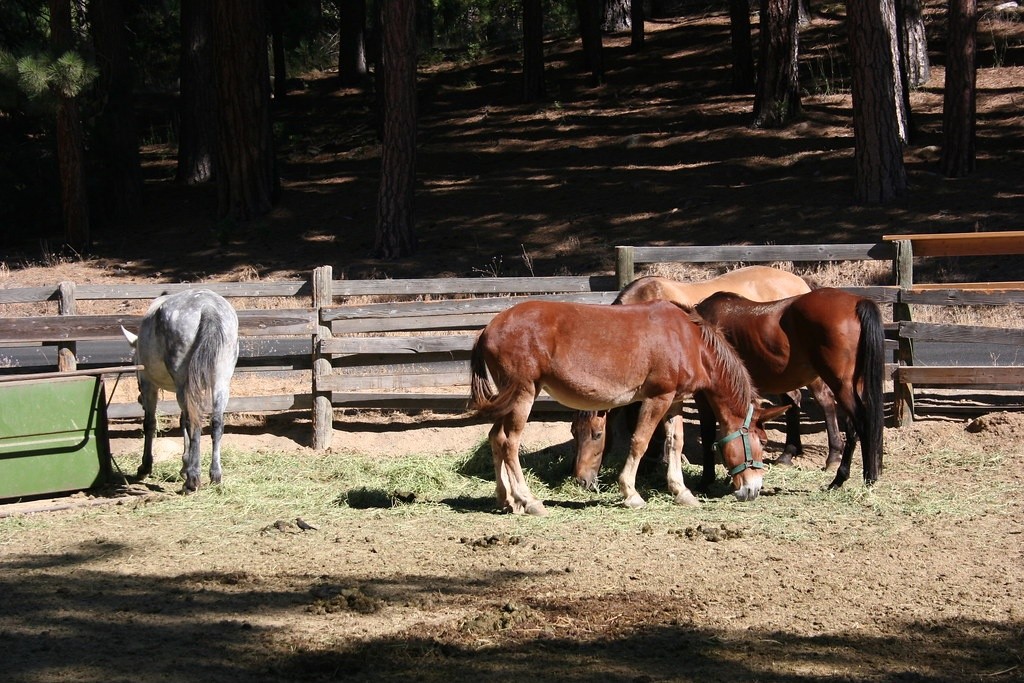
[120, 287, 240, 495]
[469, 264, 887, 518]
